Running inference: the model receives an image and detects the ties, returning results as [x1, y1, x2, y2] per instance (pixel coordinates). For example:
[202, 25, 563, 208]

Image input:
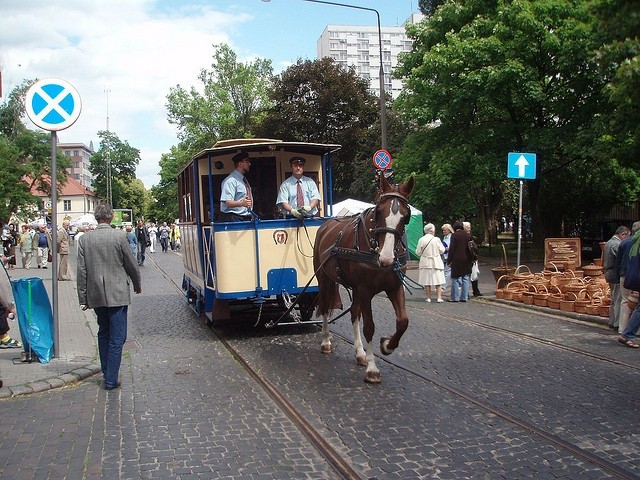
[297, 180, 304, 208]
[243, 178, 252, 207]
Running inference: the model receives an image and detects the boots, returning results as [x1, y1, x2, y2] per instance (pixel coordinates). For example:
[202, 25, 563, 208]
[470, 280, 483, 297]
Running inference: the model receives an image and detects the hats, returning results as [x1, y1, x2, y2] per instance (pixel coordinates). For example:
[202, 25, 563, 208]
[232, 152, 250, 162]
[290, 157, 306, 165]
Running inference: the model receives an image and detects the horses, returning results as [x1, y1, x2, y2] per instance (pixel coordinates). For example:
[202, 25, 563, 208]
[312, 169, 416, 384]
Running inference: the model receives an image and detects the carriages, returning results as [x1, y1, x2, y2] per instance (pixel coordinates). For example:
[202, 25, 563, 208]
[176, 137, 416, 383]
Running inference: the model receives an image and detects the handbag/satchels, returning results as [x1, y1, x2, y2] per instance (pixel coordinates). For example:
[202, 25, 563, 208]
[48, 249, 52, 262]
[468, 241, 479, 260]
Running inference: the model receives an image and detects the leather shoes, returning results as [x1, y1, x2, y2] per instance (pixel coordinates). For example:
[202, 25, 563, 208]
[437, 298, 444, 303]
[424, 298, 431, 303]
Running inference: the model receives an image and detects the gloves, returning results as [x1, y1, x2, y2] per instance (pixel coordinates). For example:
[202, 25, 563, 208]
[300, 206, 311, 216]
[291, 208, 304, 220]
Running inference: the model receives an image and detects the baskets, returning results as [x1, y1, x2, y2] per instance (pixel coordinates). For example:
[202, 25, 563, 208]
[511, 264, 534, 281]
[592, 305, 597, 315]
[495, 275, 514, 299]
[583, 276, 611, 291]
[541, 262, 561, 279]
[581, 263, 604, 276]
[598, 305, 610, 317]
[592, 291, 611, 305]
[559, 269, 581, 286]
[522, 285, 537, 304]
[575, 289, 591, 314]
[628, 291, 639, 310]
[563, 269, 584, 277]
[547, 285, 564, 309]
[512, 284, 529, 302]
[560, 292, 578, 312]
[585, 305, 592, 315]
[528, 274, 550, 290]
[503, 281, 521, 300]
[533, 284, 552, 307]
[490, 244, 516, 289]
[564, 278, 586, 300]
[551, 274, 564, 285]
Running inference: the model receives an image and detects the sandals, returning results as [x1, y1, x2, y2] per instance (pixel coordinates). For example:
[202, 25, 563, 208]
[617, 337, 640, 348]
[0, 337, 22, 348]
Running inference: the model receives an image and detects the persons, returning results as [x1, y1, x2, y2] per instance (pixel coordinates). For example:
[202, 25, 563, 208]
[169, 224, 178, 251]
[435, 224, 463, 291]
[3, 224, 19, 269]
[74, 226, 85, 259]
[125, 225, 137, 256]
[147, 223, 158, 253]
[601, 226, 631, 331]
[463, 222, 483, 300]
[159, 222, 171, 253]
[0, 224, 12, 253]
[57, 220, 71, 282]
[0, 253, 22, 348]
[33, 225, 52, 269]
[447, 220, 474, 302]
[219, 152, 254, 223]
[28, 224, 35, 239]
[78, 204, 141, 391]
[135, 220, 151, 266]
[420, 224, 445, 302]
[18, 225, 33, 270]
[276, 157, 322, 218]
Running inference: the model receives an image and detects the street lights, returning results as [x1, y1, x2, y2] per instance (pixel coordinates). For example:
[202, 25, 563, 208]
[262, 0, 387, 150]
